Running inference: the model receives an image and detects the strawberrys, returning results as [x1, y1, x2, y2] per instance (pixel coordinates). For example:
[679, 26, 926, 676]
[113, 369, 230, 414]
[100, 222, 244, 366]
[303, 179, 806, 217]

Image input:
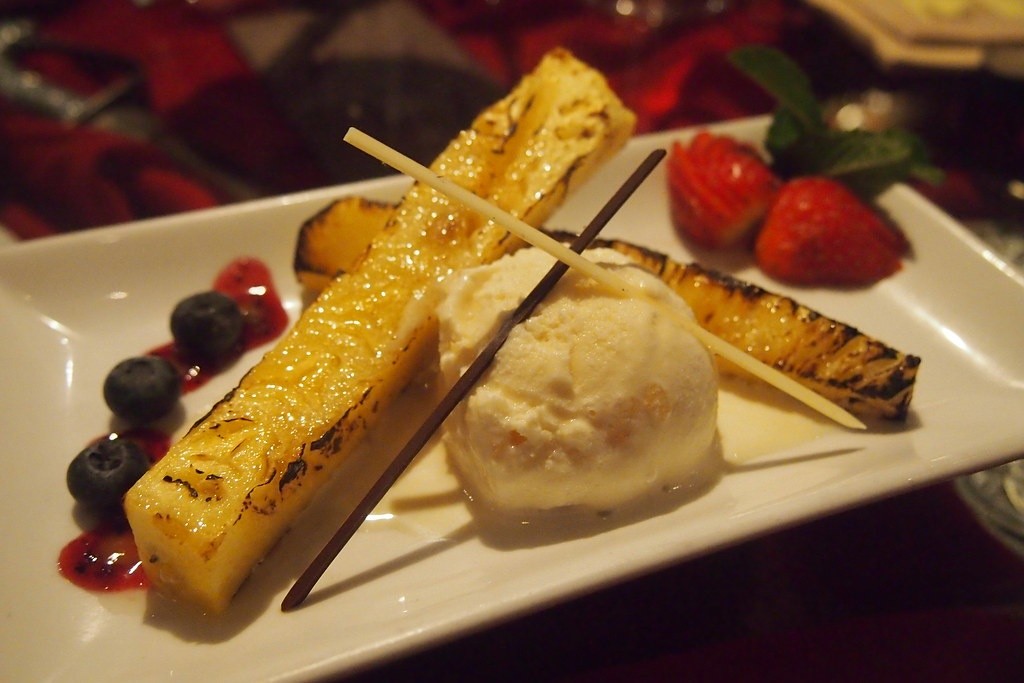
[751, 174, 909, 289]
[665, 131, 776, 253]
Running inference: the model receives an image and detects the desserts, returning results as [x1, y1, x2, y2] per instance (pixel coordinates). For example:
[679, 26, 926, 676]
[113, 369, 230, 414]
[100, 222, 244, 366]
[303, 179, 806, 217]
[433, 240, 720, 529]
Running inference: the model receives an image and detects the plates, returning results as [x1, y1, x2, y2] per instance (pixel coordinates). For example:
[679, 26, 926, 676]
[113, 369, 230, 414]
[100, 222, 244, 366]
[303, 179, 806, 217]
[1, 115, 1024, 683]
